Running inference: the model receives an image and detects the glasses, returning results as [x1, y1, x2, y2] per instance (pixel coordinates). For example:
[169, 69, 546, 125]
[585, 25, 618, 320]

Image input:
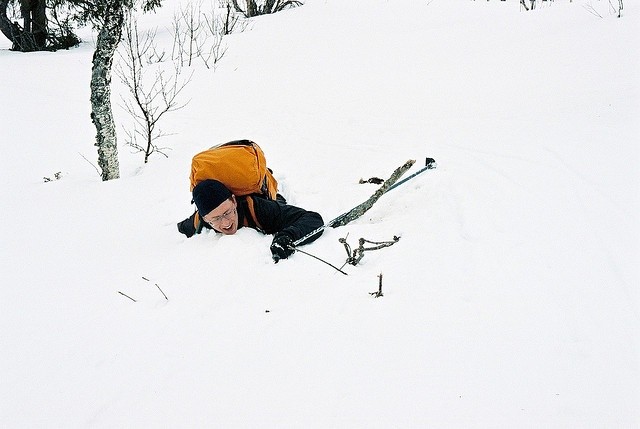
[206, 206, 236, 226]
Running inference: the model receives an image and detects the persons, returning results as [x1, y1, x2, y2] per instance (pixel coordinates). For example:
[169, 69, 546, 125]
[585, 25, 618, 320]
[176, 179, 324, 262]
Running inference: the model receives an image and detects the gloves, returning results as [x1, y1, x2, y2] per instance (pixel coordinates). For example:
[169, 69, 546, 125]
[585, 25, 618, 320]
[269, 232, 295, 264]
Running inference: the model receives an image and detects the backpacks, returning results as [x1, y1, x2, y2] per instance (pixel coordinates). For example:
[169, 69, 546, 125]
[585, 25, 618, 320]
[189, 140, 278, 230]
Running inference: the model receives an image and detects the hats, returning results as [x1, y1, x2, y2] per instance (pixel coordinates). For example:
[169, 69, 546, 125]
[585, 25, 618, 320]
[193, 179, 233, 217]
